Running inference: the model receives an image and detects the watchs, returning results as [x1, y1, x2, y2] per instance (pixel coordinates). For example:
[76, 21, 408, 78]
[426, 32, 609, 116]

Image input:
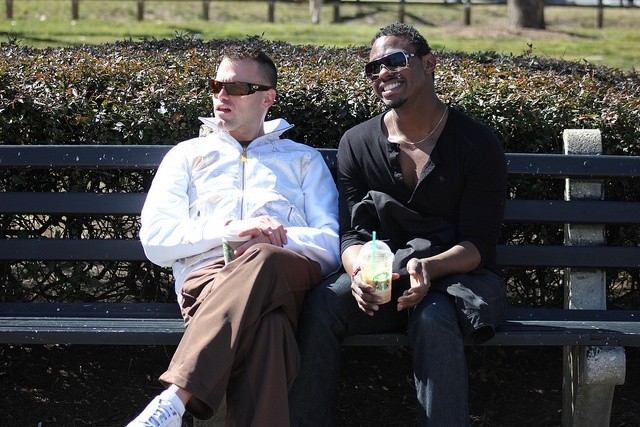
[351, 268, 361, 281]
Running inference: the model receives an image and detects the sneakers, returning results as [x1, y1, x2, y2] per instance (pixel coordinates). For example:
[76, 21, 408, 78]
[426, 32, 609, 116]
[125, 396, 182, 427]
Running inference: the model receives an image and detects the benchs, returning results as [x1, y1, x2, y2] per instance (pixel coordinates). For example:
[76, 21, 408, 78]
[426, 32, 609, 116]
[0, 128, 640, 427]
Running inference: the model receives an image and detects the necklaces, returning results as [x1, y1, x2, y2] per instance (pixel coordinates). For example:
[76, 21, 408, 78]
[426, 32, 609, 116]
[391, 106, 448, 145]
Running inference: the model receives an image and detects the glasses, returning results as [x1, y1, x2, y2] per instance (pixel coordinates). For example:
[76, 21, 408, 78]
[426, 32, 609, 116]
[208, 79, 274, 95]
[365, 50, 419, 80]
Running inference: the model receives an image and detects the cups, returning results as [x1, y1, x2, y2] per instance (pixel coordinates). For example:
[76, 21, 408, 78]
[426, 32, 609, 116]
[356, 230, 395, 305]
[222, 230, 252, 266]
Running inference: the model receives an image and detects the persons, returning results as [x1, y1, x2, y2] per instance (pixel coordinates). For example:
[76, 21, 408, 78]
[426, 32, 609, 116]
[125, 50, 342, 426]
[288, 23, 508, 427]
